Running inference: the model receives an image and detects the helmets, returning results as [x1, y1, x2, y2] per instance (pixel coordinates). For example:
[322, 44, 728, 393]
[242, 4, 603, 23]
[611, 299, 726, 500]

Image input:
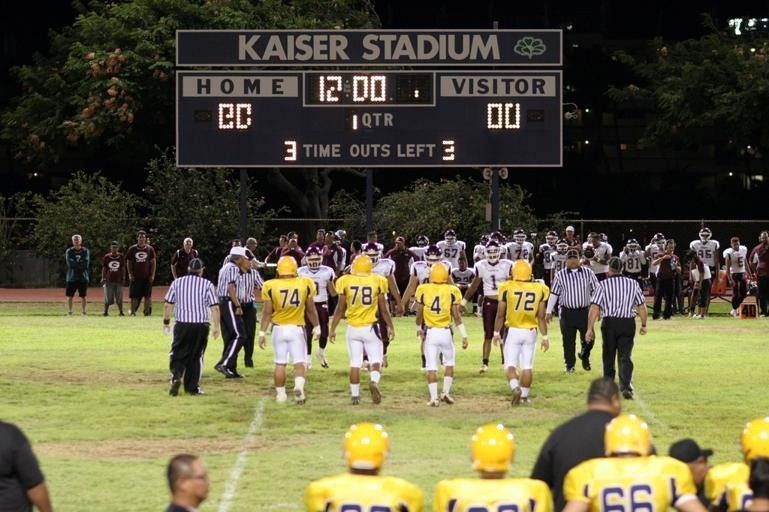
[414, 226, 669, 272]
[697, 226, 712, 243]
[425, 260, 449, 285]
[275, 254, 299, 277]
[600, 413, 654, 459]
[469, 420, 520, 476]
[734, 414, 769, 461]
[363, 242, 379, 262]
[305, 243, 324, 274]
[341, 418, 390, 474]
[351, 251, 375, 277]
[506, 259, 534, 282]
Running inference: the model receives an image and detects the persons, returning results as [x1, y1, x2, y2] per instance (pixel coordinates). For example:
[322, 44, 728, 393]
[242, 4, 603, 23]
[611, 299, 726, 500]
[459, 239, 514, 373]
[545, 249, 601, 372]
[257, 255, 322, 405]
[402, 245, 453, 370]
[237, 247, 265, 367]
[343, 243, 402, 370]
[297, 245, 338, 369]
[493, 259, 549, 405]
[415, 261, 468, 406]
[585, 256, 648, 399]
[2, 417, 53, 512]
[328, 254, 395, 404]
[214, 244, 250, 378]
[165, 258, 220, 397]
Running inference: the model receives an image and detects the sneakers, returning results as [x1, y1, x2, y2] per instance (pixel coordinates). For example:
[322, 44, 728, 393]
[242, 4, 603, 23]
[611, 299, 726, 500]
[65, 310, 153, 318]
[479, 365, 489, 374]
[519, 396, 535, 405]
[652, 310, 769, 320]
[274, 392, 290, 403]
[293, 386, 306, 406]
[315, 351, 330, 368]
[426, 397, 439, 408]
[306, 354, 313, 370]
[351, 394, 360, 405]
[439, 391, 457, 405]
[367, 379, 383, 405]
[510, 386, 521, 409]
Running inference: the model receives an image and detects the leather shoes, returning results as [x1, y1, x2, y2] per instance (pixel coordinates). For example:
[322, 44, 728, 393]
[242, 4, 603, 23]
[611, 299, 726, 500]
[225, 370, 244, 379]
[167, 376, 181, 397]
[621, 389, 634, 401]
[577, 350, 591, 371]
[184, 386, 206, 396]
[563, 364, 574, 373]
[213, 362, 234, 376]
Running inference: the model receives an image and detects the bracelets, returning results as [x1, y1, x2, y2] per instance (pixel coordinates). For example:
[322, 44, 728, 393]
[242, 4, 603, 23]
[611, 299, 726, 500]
[259, 330, 266, 337]
[494, 331, 500, 336]
[460, 297, 467, 305]
[164, 318, 170, 325]
[416, 323, 422, 332]
[642, 324, 647, 328]
[458, 324, 467, 339]
[543, 335, 548, 341]
[236, 304, 241, 308]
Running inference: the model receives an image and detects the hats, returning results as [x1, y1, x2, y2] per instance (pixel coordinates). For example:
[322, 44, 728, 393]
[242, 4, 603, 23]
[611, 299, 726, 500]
[229, 246, 249, 259]
[393, 236, 405, 244]
[666, 435, 719, 463]
[187, 257, 206, 272]
